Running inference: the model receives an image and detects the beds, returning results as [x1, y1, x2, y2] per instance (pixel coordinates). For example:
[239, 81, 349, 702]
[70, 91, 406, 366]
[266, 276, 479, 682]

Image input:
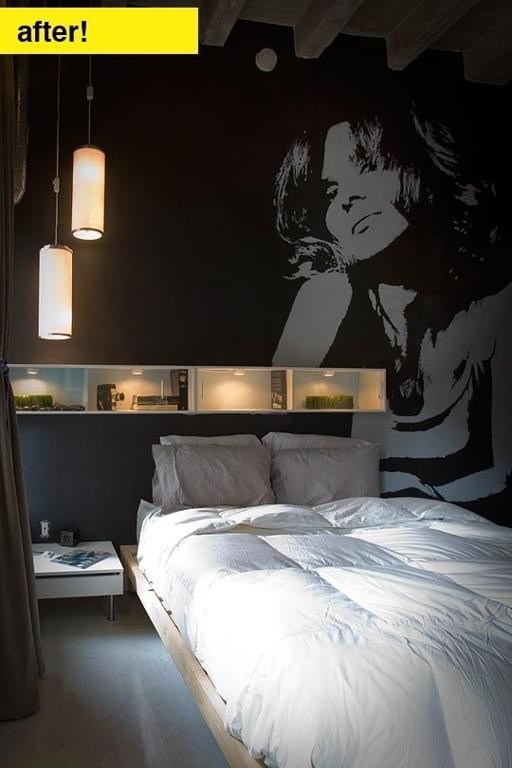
[115, 502, 512, 768]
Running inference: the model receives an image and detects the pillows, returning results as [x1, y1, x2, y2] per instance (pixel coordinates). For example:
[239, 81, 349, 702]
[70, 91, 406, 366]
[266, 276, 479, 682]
[145, 428, 386, 513]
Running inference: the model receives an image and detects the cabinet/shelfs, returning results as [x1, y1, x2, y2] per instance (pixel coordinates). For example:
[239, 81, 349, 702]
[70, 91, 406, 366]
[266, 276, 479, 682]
[5, 363, 389, 416]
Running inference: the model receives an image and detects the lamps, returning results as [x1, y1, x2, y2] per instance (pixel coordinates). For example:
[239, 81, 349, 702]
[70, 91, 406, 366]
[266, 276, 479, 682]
[35, 43, 109, 342]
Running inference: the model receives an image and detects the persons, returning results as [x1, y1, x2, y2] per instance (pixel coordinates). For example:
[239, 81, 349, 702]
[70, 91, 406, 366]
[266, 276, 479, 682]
[270, 93, 512, 527]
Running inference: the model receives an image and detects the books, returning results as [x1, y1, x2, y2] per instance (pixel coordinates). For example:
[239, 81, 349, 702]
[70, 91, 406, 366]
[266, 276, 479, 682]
[50, 548, 112, 570]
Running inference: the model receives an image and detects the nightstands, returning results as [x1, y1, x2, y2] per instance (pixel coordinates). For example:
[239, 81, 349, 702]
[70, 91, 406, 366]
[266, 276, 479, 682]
[27, 540, 125, 624]
